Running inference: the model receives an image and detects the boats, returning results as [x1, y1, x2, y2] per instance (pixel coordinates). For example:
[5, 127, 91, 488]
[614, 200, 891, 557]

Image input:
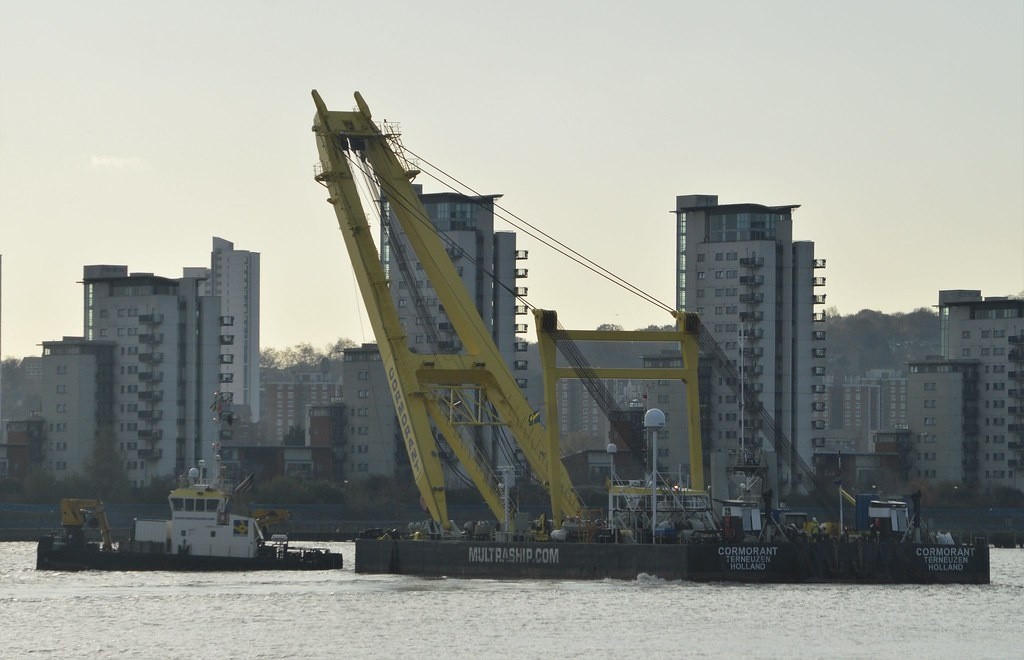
[36, 389, 342, 572]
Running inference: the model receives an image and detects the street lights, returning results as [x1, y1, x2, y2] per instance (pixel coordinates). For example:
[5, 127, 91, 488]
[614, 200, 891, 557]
[644, 409, 665, 544]
[606, 443, 617, 528]
